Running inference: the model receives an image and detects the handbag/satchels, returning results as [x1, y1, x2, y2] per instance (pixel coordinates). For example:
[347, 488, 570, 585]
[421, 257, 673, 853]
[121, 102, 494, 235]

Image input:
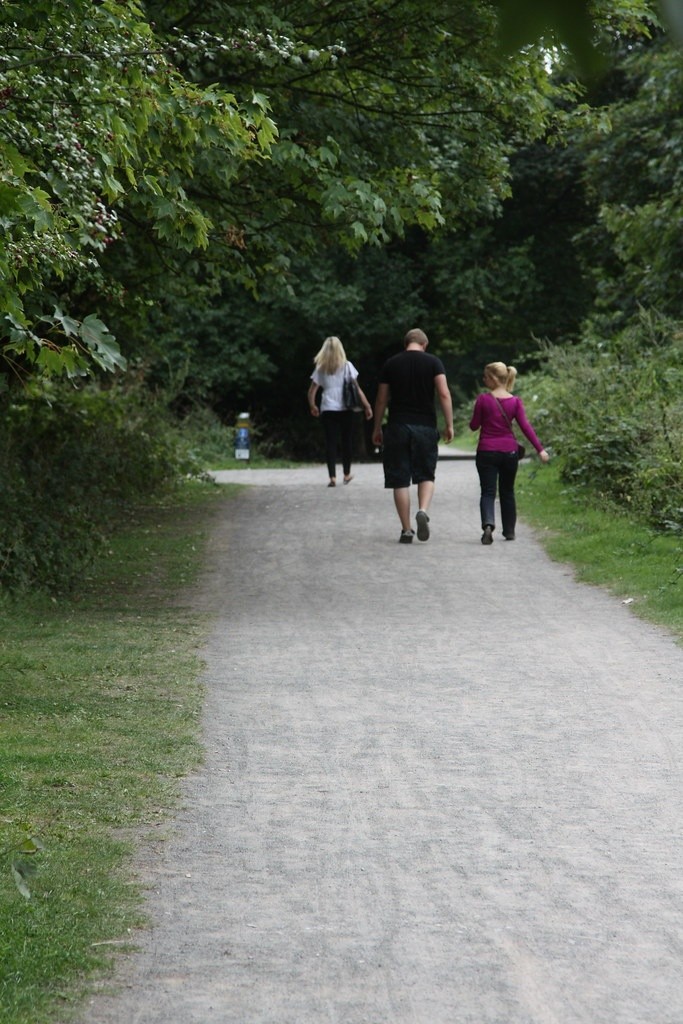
[518, 443, 525, 460]
[343, 362, 358, 408]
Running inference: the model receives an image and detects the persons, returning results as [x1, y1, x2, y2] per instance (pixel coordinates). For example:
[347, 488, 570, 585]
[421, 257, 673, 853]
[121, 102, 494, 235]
[373, 329, 455, 543]
[469, 361, 549, 546]
[308, 337, 373, 488]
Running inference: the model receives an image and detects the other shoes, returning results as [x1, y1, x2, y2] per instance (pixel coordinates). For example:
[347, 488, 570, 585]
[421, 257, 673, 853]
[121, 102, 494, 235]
[481, 526, 493, 545]
[415, 511, 429, 541]
[506, 534, 514, 540]
[400, 529, 415, 543]
[344, 474, 353, 484]
[328, 482, 335, 487]
